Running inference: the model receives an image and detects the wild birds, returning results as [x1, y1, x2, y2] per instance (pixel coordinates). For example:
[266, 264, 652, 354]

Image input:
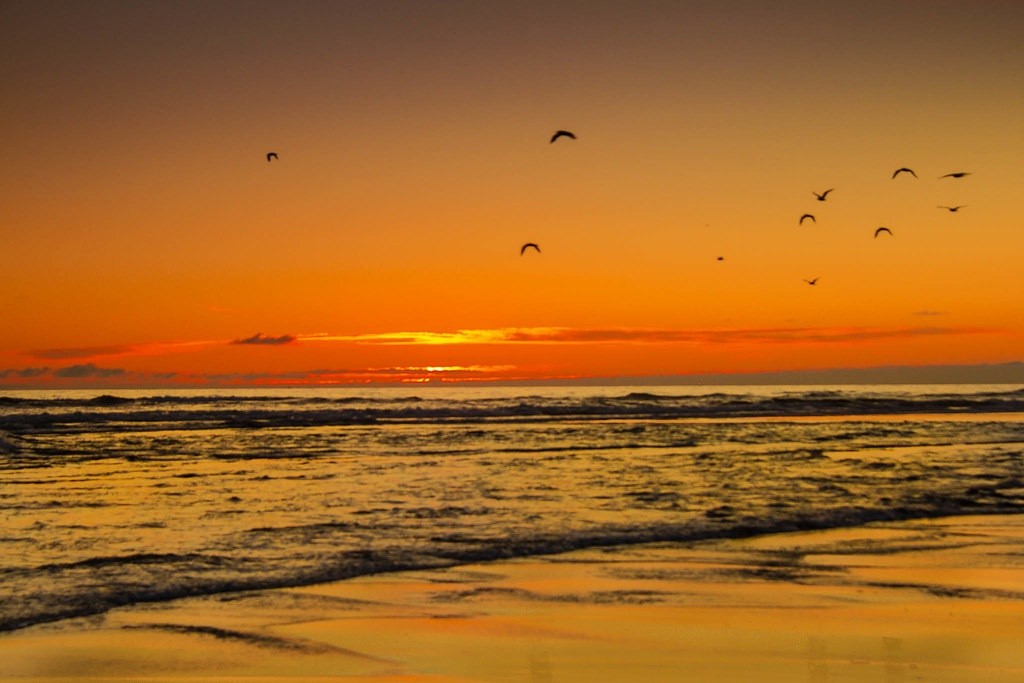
[892, 168, 917, 179]
[521, 243, 540, 255]
[812, 189, 833, 201]
[266, 152, 279, 161]
[551, 130, 577, 144]
[943, 173, 971, 178]
[799, 214, 816, 225]
[937, 205, 965, 212]
[803, 276, 819, 285]
[874, 227, 893, 239]
[717, 257, 725, 262]
